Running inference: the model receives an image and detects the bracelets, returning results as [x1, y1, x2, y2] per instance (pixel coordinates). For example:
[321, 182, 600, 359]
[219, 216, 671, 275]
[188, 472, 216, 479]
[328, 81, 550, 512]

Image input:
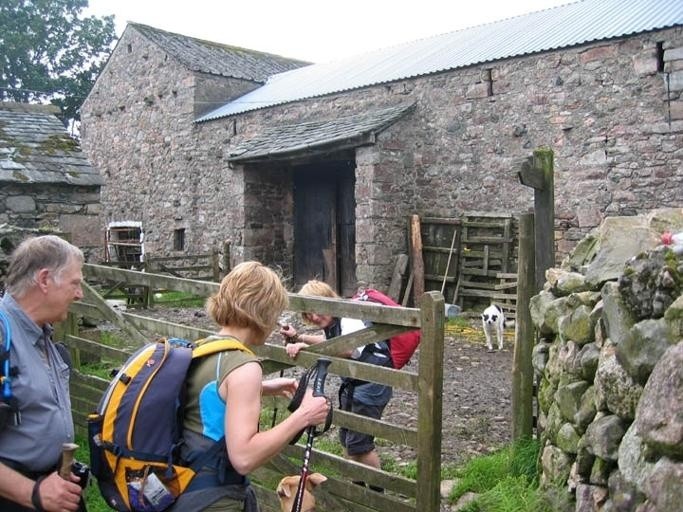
[286, 333, 299, 343]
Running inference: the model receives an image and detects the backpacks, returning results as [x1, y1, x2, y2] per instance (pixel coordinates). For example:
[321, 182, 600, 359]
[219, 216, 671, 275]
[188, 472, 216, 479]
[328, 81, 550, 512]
[335, 287, 420, 373]
[88, 336, 258, 512]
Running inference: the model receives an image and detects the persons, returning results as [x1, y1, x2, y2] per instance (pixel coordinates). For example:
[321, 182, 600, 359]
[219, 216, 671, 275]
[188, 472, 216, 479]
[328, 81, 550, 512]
[172, 260, 328, 512]
[280, 280, 394, 495]
[0, 235, 87, 511]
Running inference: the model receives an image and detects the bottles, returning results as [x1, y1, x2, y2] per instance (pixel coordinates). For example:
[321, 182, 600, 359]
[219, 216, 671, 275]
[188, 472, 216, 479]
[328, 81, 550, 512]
[661, 230, 682, 249]
[87, 413, 104, 477]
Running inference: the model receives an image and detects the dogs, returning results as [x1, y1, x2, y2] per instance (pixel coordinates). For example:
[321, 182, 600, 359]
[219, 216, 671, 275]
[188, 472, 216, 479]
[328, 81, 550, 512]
[275, 472, 328, 512]
[479, 303, 508, 351]
